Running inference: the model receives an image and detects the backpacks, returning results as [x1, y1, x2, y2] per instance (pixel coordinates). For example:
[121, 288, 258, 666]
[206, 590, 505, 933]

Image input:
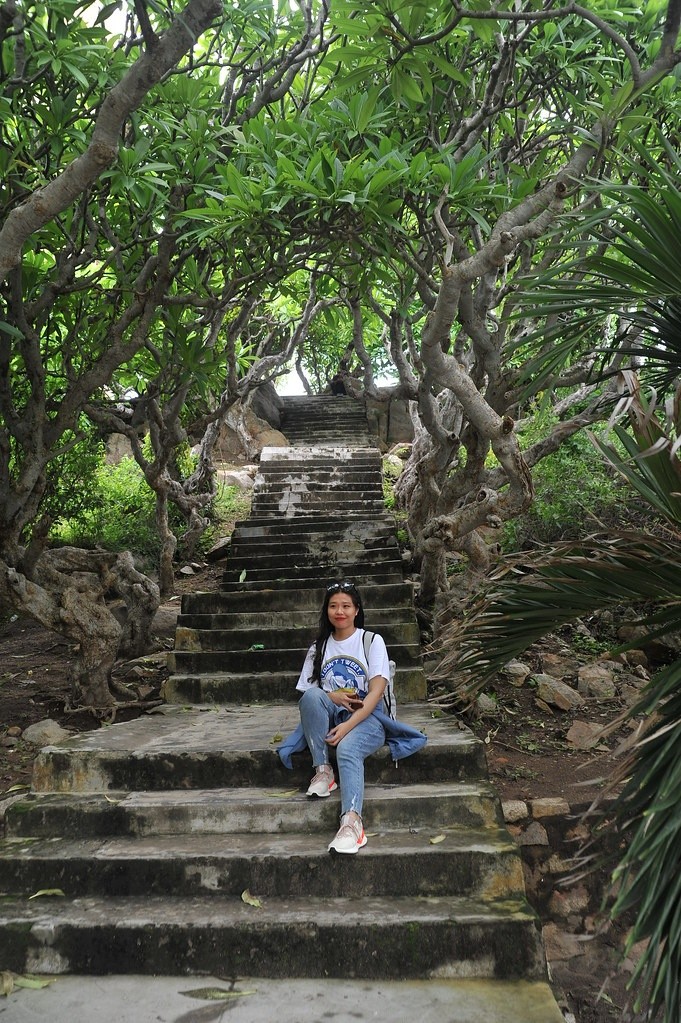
[363, 630, 396, 721]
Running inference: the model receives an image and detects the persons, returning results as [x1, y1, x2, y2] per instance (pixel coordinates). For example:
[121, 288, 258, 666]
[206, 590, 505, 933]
[296, 582, 389, 854]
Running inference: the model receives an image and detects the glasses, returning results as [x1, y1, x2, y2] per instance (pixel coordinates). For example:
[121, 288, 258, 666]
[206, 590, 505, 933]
[325, 580, 356, 593]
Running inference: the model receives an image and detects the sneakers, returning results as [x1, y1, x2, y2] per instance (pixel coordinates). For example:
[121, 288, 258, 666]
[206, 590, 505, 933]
[305, 764, 338, 797]
[326, 815, 368, 854]
